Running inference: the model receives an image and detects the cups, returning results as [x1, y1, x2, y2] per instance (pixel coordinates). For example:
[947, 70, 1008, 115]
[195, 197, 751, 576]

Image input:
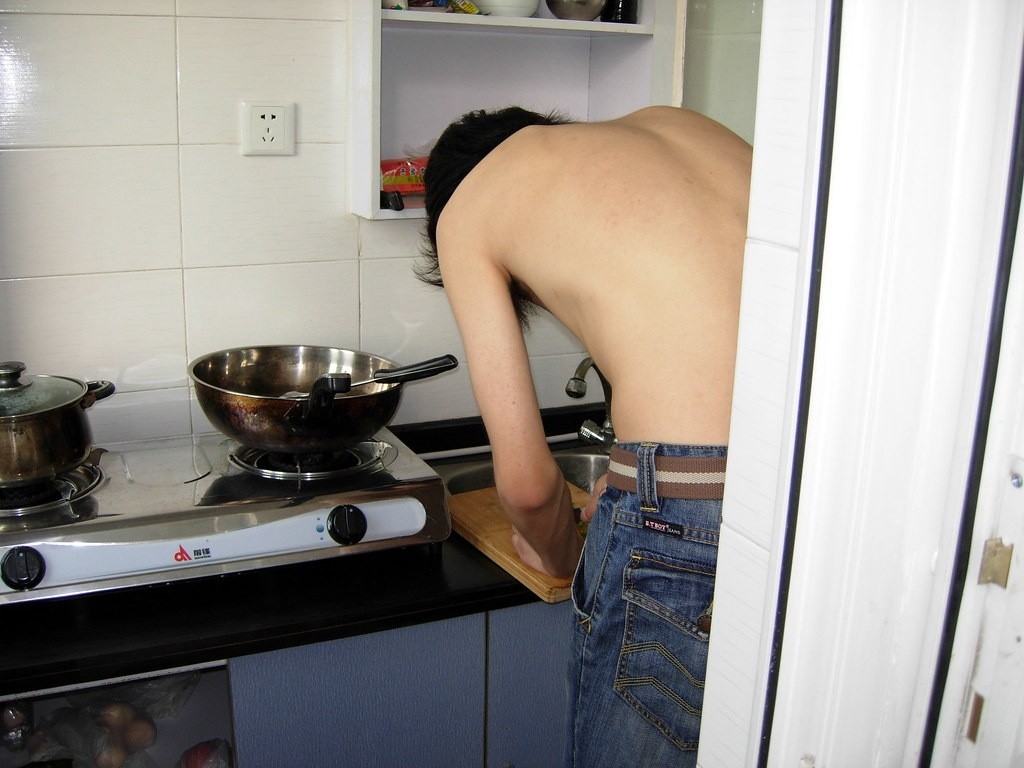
[600, 0, 637, 24]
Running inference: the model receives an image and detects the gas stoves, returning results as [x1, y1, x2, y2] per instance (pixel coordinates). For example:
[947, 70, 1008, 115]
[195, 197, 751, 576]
[0, 435, 452, 607]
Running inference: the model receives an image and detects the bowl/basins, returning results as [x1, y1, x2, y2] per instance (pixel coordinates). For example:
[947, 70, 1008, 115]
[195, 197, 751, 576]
[545, 0, 606, 21]
[472, 0, 539, 17]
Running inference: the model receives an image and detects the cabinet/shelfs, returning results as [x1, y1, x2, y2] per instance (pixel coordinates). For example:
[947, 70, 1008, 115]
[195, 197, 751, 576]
[340, 1, 687, 219]
[0, 594, 581, 768]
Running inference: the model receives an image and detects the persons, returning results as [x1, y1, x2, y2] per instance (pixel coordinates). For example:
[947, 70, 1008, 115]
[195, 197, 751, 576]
[410, 105, 753, 768]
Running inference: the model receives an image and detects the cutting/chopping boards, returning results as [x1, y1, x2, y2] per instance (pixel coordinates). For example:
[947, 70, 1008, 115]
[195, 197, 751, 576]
[447, 480, 600, 604]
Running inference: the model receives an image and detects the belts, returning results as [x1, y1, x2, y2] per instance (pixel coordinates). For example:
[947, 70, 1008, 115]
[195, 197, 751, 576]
[604, 448, 726, 501]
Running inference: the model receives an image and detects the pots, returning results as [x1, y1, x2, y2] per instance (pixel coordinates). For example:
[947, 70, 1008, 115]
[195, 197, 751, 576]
[0, 358, 115, 486]
[187, 343, 459, 458]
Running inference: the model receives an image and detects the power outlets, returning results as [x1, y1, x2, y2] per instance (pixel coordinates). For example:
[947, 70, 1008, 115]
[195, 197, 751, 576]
[240, 100, 296, 157]
